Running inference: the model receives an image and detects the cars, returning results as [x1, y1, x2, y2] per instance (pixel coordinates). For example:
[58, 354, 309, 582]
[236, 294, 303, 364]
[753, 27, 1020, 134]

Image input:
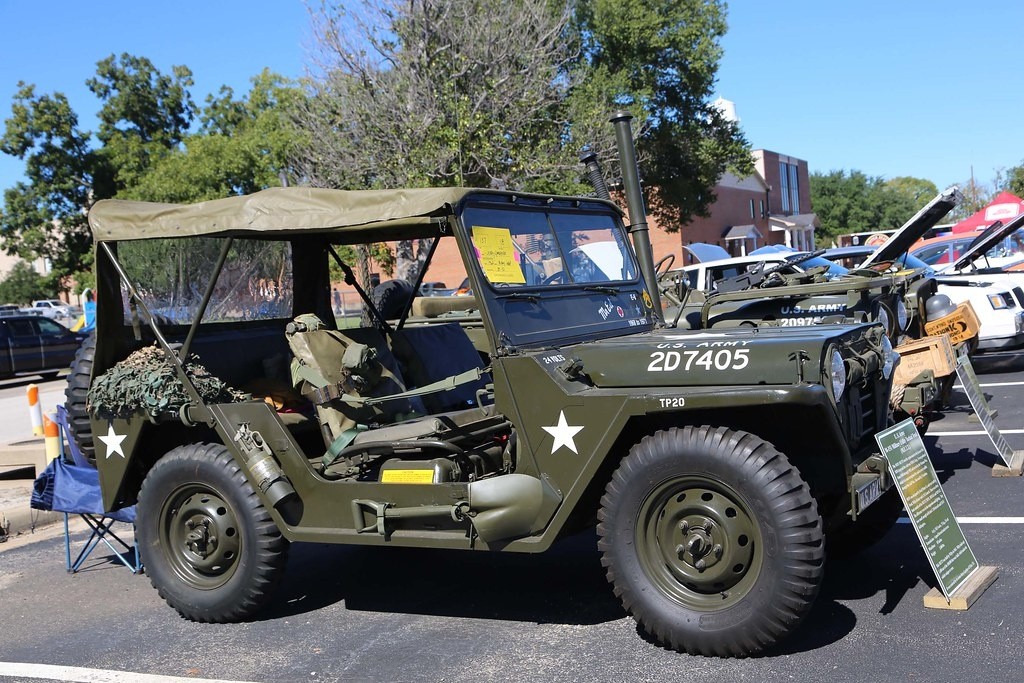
[566, 187, 1024, 437]
[31, 299, 76, 320]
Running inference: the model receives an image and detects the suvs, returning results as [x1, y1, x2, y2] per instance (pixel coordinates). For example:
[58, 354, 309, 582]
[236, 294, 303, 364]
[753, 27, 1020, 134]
[1, 309, 88, 379]
[65, 108, 930, 660]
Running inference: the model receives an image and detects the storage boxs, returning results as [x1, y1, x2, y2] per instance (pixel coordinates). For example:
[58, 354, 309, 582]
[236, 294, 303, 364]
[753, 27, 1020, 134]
[890, 332, 958, 386]
[924, 299, 982, 345]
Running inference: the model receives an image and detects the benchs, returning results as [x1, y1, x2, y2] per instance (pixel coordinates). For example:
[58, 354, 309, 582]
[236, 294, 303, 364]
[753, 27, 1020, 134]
[147, 328, 318, 435]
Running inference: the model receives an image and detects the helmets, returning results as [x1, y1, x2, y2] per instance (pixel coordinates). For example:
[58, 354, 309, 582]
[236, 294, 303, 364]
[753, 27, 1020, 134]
[925, 294, 956, 321]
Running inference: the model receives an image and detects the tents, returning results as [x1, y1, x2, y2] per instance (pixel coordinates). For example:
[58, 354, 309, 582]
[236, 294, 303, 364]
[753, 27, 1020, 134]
[952, 191, 1024, 258]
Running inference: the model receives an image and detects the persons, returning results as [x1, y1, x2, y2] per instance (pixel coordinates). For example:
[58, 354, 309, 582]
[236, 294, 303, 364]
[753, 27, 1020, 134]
[799, 241, 802, 251]
[334, 288, 342, 314]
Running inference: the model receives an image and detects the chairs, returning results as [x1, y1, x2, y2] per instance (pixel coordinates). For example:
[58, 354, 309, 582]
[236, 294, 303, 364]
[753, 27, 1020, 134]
[284, 326, 504, 483]
[392, 320, 493, 412]
[29, 405, 144, 575]
